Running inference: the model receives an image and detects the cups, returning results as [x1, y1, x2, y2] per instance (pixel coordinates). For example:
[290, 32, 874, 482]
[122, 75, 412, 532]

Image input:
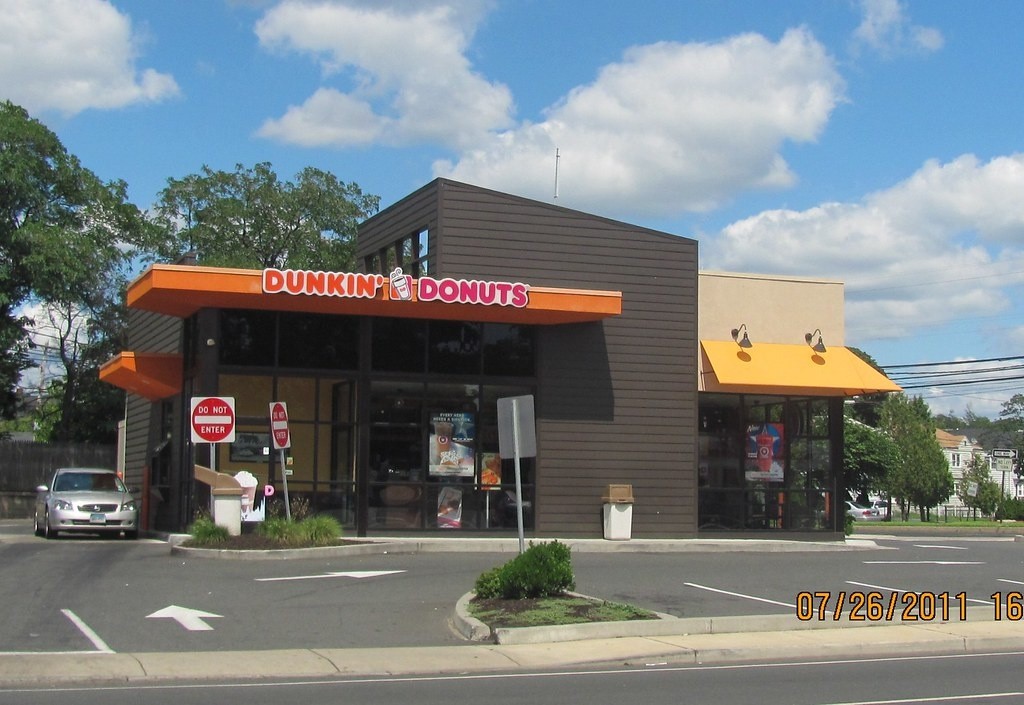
[756, 434, 773, 471]
[393, 276, 410, 299]
[434, 422, 455, 456]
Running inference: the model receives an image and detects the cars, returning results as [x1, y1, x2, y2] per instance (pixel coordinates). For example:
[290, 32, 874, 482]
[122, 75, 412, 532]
[869, 499, 888, 517]
[844, 500, 880, 524]
[34, 468, 140, 540]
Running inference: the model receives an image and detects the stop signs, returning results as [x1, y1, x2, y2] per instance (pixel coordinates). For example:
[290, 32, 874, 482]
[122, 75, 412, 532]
[269, 401, 291, 449]
[190, 396, 235, 443]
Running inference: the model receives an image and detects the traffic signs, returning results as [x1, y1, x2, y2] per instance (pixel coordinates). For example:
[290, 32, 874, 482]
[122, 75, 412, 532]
[992, 449, 1018, 459]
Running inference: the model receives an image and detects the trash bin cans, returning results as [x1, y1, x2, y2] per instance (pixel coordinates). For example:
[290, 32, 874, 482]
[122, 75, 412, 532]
[601, 484, 633, 541]
[212, 473, 243, 536]
